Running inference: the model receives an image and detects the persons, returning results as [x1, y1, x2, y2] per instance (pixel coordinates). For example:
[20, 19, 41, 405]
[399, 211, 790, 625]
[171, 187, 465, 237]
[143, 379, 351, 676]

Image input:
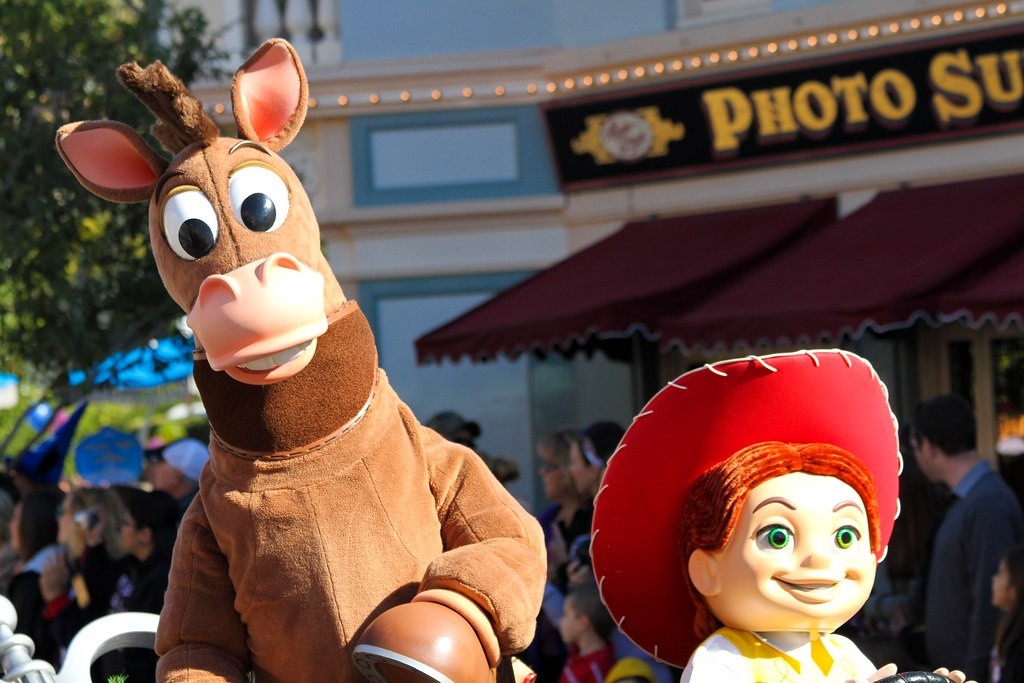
[0, 437, 210, 683]
[838, 391, 1024, 683]
[426, 410, 681, 683]
[586, 350, 976, 683]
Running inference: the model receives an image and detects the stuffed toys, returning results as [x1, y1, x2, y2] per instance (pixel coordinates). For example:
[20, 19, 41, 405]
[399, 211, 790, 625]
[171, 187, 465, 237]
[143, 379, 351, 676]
[57, 38, 547, 683]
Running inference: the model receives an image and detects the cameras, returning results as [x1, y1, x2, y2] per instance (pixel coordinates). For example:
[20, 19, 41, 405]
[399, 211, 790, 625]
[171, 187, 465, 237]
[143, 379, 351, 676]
[74, 510, 97, 531]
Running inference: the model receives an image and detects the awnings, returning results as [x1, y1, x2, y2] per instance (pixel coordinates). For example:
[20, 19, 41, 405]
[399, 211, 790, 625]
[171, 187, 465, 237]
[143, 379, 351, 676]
[415, 171, 1023, 365]
